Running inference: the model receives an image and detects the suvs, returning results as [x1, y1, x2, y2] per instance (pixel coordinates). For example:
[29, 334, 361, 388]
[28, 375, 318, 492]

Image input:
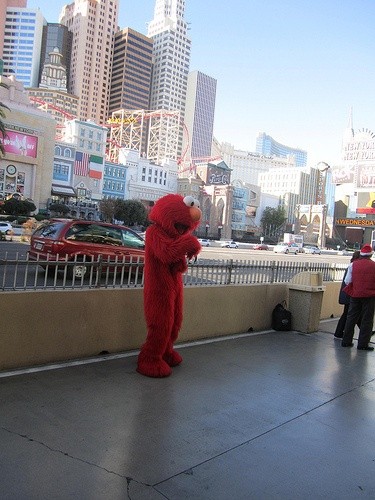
[26, 217, 155, 280]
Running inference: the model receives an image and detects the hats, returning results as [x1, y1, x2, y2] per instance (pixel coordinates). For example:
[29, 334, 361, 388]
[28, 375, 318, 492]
[360, 244, 374, 256]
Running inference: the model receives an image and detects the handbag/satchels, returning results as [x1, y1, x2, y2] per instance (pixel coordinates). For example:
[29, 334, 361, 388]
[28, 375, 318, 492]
[271, 299, 292, 331]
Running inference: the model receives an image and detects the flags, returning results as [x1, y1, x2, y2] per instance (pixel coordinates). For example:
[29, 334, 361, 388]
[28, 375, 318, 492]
[74, 150, 104, 179]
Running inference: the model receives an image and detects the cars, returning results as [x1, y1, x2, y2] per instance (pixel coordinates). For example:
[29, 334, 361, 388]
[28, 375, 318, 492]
[302, 244, 322, 255]
[251, 244, 269, 250]
[0, 222, 14, 236]
[273, 242, 300, 255]
[220, 240, 239, 249]
[343, 248, 357, 256]
[196, 238, 211, 247]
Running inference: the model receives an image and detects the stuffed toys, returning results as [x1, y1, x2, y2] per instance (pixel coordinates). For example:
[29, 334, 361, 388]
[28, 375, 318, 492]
[136, 194, 202, 379]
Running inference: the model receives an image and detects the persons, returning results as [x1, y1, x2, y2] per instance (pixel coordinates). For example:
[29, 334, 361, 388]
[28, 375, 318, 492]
[341, 244, 375, 351]
[334, 251, 375, 339]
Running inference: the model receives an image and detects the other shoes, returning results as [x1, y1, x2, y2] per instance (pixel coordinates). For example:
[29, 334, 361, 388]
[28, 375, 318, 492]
[357, 345, 374, 350]
[341, 342, 353, 347]
[371, 330, 375, 336]
[334, 332, 344, 338]
